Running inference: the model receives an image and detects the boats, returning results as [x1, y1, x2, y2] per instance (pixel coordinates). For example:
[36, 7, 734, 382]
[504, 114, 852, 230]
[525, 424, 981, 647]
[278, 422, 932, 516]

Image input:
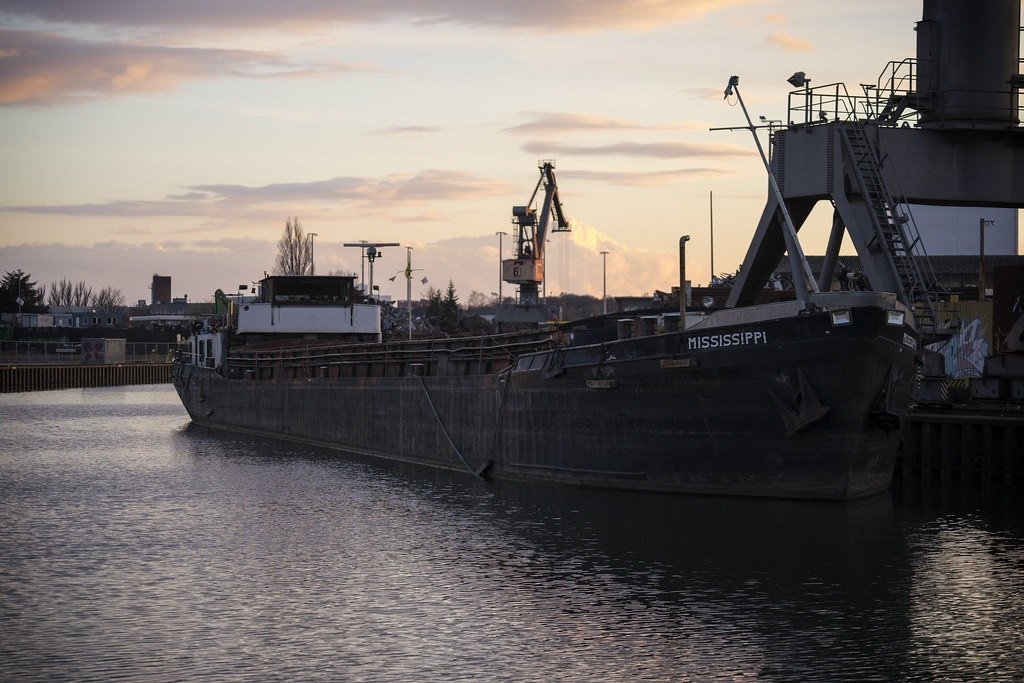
[167, 233, 917, 548]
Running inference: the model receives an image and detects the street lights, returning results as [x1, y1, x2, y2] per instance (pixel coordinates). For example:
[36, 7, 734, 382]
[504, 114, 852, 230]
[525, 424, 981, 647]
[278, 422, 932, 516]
[308, 233, 317, 275]
[496, 232, 507, 306]
[359, 239, 369, 294]
[404, 246, 414, 315]
[599, 251, 611, 314]
